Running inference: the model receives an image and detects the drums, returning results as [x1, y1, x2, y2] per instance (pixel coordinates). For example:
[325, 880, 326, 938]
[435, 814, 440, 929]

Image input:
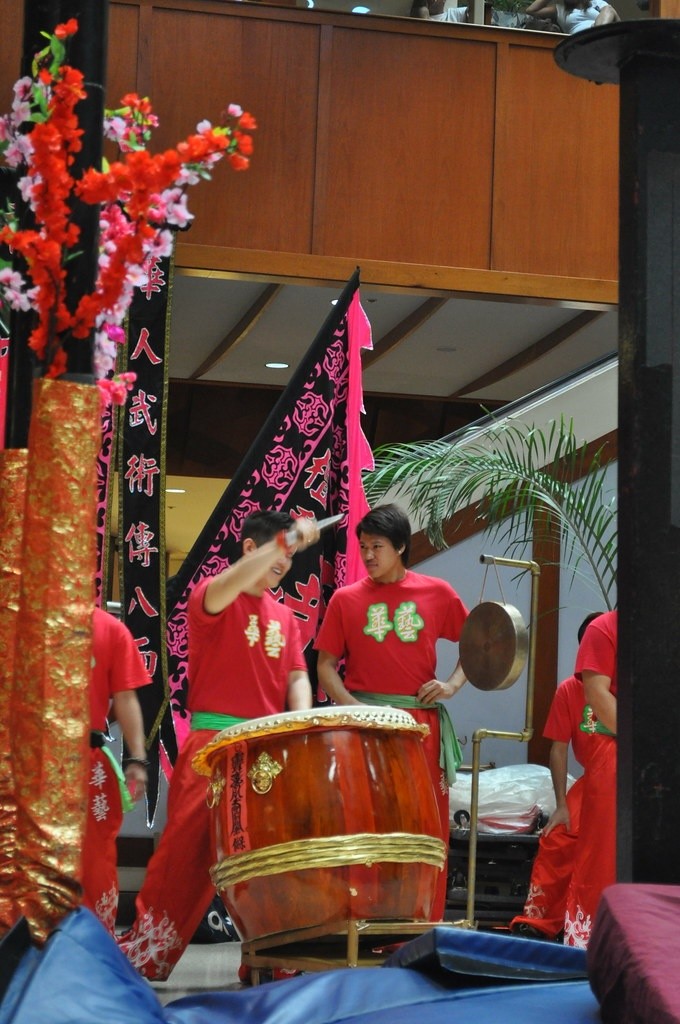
[189, 704, 451, 944]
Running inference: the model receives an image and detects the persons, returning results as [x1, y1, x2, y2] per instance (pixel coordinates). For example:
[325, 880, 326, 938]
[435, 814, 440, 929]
[311, 504, 470, 953]
[410, 0, 468, 22]
[525, 0, 622, 34]
[81, 609, 152, 940]
[510, 612, 618, 950]
[119, 511, 322, 983]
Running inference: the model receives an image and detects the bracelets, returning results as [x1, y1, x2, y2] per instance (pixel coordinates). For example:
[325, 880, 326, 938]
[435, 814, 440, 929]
[122, 759, 151, 768]
[278, 532, 289, 550]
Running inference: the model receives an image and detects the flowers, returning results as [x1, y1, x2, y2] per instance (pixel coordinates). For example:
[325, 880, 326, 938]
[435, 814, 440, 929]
[0, 17, 258, 388]
